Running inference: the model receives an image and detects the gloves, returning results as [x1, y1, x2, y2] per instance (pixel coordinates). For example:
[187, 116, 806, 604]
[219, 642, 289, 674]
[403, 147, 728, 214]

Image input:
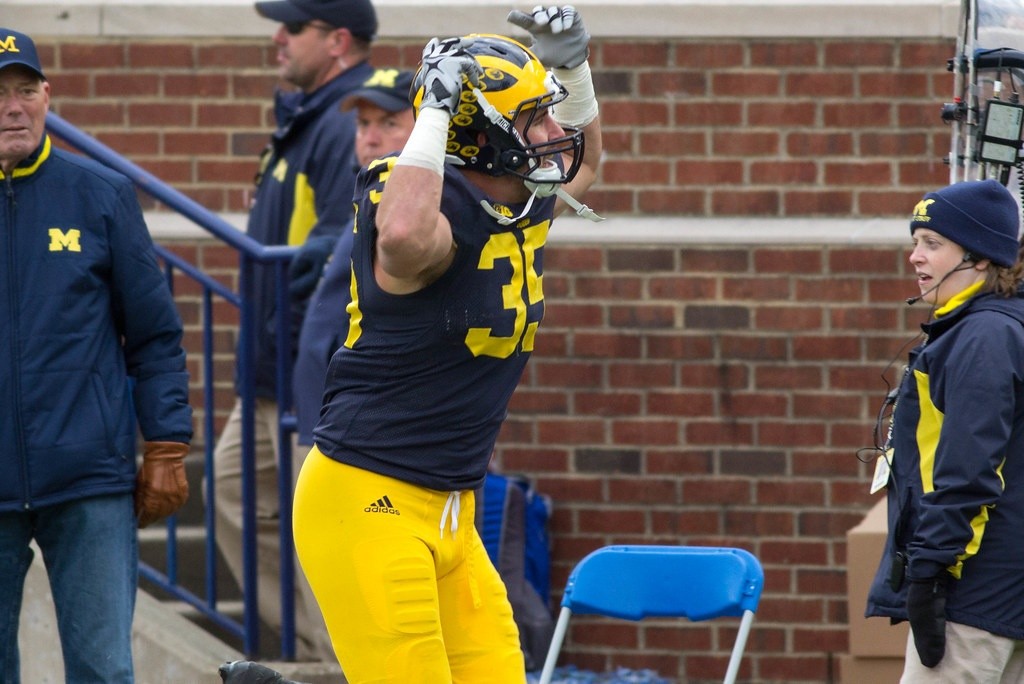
[135, 441, 188, 530]
[907, 581, 948, 669]
[507, 5, 591, 70]
[419, 35, 485, 117]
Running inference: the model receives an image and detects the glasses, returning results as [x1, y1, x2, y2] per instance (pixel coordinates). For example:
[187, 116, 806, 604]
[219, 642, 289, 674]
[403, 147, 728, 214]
[283, 19, 333, 35]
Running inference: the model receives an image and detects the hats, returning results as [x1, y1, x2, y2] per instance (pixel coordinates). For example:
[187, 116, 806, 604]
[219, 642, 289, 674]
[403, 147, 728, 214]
[255, 0, 377, 38]
[0, 27, 46, 80]
[338, 66, 418, 112]
[910, 180, 1020, 268]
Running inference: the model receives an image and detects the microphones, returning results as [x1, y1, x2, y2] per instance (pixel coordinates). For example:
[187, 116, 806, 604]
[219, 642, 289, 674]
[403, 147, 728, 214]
[906, 261, 965, 306]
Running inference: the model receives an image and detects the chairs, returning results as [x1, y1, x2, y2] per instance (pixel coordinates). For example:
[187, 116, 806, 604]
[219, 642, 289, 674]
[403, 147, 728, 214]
[539, 544, 765, 684]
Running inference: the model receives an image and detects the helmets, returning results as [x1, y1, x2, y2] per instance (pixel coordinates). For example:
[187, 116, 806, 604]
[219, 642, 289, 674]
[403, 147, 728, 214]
[409, 32, 584, 199]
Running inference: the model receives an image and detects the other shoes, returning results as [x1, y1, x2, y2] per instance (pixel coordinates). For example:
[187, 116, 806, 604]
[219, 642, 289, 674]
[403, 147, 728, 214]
[217, 659, 281, 684]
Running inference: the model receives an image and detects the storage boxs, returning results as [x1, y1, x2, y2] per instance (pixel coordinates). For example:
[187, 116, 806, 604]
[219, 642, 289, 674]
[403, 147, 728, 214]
[837, 498, 910, 684]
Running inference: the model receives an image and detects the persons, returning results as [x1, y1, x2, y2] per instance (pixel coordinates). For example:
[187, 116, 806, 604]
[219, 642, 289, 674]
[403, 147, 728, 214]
[292, 6, 601, 684]
[201, 0, 378, 660]
[292, 70, 420, 488]
[865, 180, 1024, 684]
[0, 28, 195, 684]
